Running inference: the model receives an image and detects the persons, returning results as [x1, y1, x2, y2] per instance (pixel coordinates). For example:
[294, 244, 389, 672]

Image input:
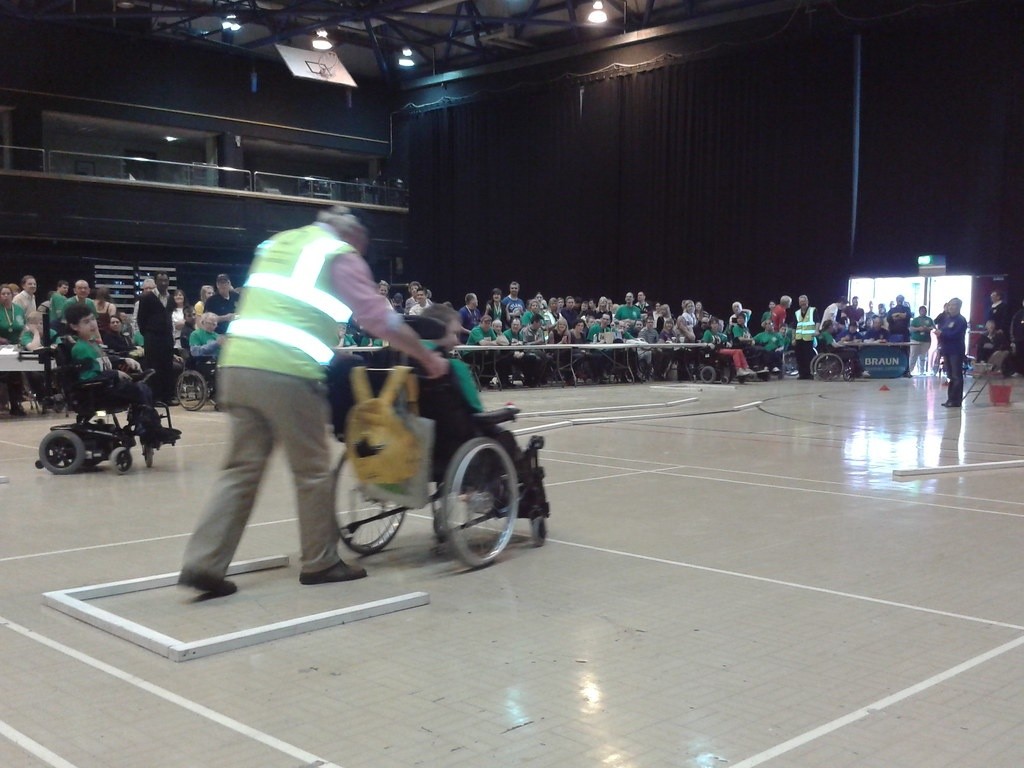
[334, 279, 1024, 407]
[179, 207, 448, 596]
[0, 273, 243, 415]
[419, 305, 516, 427]
[64, 302, 176, 448]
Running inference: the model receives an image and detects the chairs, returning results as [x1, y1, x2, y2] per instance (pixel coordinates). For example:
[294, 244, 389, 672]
[962, 351, 1008, 406]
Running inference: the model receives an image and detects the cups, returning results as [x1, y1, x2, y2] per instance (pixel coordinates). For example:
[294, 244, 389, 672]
[680, 337, 685, 343]
[604, 332, 614, 343]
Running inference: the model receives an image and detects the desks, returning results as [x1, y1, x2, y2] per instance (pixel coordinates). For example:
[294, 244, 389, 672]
[842, 340, 921, 379]
[335, 343, 706, 392]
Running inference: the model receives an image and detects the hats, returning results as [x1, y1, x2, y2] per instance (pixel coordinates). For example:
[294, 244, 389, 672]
[217, 274, 229, 281]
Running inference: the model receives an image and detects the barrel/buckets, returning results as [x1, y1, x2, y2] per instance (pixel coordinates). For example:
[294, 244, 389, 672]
[989, 384, 1012, 405]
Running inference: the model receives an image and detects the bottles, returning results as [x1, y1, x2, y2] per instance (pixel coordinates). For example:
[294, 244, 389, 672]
[593, 333, 597, 344]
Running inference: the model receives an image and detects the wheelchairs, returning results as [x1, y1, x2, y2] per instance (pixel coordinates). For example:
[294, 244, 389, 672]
[700, 342, 749, 385]
[810, 334, 861, 382]
[176, 355, 226, 413]
[18, 312, 182, 476]
[753, 348, 785, 382]
[783, 345, 818, 377]
[325, 342, 551, 570]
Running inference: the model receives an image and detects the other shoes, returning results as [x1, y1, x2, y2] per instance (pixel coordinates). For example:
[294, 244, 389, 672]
[177, 569, 238, 595]
[154, 401, 165, 407]
[299, 559, 367, 585]
[861, 371, 870, 377]
[164, 399, 179, 406]
[746, 369, 756, 375]
[785, 369, 799, 376]
[502, 383, 517, 388]
[737, 368, 749, 377]
[941, 400, 961, 407]
[796, 376, 810, 379]
[139, 425, 182, 444]
[764, 367, 768, 370]
[523, 380, 539, 388]
[773, 367, 780, 371]
[9, 408, 26, 416]
